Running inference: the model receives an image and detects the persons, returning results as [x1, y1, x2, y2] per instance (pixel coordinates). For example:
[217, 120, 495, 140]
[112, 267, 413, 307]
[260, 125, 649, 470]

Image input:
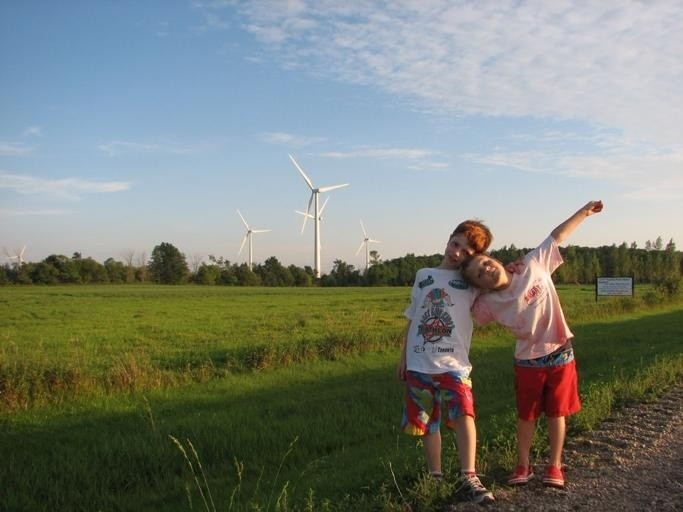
[463, 200, 604, 488]
[398, 220, 495, 507]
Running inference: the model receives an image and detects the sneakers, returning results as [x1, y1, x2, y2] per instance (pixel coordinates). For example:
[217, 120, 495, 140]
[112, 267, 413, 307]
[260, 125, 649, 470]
[462, 470, 496, 506]
[542, 464, 565, 488]
[508, 465, 534, 485]
